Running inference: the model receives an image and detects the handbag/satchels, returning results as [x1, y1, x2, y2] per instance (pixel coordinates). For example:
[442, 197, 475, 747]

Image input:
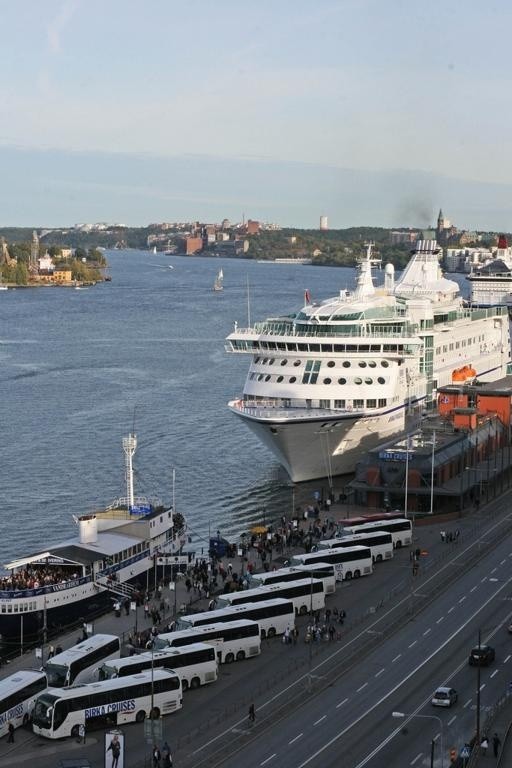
[481, 740, 488, 749]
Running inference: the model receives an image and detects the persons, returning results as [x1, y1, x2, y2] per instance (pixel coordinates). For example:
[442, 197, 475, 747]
[248, 703, 255, 720]
[441, 527, 462, 543]
[451, 733, 500, 767]
[282, 607, 345, 646]
[49, 630, 88, 658]
[107, 736, 121, 767]
[185, 491, 346, 596]
[8, 721, 15, 742]
[1, 559, 117, 591]
[112, 567, 215, 654]
[470, 489, 485, 509]
[410, 547, 421, 561]
[383, 501, 429, 512]
[153, 743, 174, 767]
[157, 506, 185, 532]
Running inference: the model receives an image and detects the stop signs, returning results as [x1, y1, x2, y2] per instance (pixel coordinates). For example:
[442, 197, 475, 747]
[420, 550, 429, 558]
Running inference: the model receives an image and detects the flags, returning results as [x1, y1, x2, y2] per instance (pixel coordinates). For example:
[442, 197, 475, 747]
[305, 288, 310, 304]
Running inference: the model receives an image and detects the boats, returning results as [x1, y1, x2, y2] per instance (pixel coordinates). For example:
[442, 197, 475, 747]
[224, 239, 512, 484]
[1, 431, 188, 641]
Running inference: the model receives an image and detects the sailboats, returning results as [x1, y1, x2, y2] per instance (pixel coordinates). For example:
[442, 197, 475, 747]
[214, 268, 224, 291]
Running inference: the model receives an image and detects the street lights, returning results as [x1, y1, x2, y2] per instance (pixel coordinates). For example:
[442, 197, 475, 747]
[391, 711, 443, 768]
[125, 643, 180, 749]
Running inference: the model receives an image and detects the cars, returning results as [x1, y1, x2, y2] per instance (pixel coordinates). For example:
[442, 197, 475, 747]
[431, 687, 457, 708]
[469, 645, 495, 667]
